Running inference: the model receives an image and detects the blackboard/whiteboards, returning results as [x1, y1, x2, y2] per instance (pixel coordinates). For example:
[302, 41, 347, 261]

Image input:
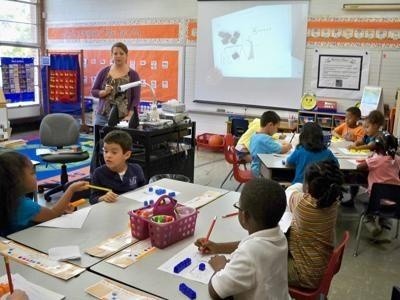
[183, 45, 400, 124]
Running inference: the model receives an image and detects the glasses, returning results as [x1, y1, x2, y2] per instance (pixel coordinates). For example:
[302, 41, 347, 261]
[233, 202, 248, 214]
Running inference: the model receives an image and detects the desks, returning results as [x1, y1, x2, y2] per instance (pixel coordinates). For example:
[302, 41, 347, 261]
[6, 178, 229, 270]
[267, 132, 360, 153]
[90, 190, 292, 300]
[0, 236, 159, 300]
[256, 153, 369, 212]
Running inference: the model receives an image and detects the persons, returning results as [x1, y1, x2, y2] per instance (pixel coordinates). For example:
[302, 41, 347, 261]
[285, 122, 339, 184]
[353, 133, 400, 244]
[90, 42, 141, 172]
[90, 130, 147, 203]
[235, 118, 286, 162]
[0, 152, 90, 237]
[285, 161, 344, 290]
[248, 111, 293, 178]
[340, 111, 397, 208]
[6, 290, 29, 300]
[193, 179, 289, 300]
[331, 107, 366, 142]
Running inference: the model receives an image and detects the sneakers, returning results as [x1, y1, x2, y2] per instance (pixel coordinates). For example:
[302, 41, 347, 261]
[364, 223, 381, 233]
[375, 227, 392, 243]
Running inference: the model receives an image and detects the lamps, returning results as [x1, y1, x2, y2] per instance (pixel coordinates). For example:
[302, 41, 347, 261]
[343, 3, 400, 11]
[117, 81, 158, 123]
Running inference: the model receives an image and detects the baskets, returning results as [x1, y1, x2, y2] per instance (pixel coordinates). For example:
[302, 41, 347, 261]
[128, 195, 197, 249]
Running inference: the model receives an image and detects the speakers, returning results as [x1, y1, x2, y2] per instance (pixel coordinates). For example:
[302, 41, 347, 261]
[231, 119, 248, 137]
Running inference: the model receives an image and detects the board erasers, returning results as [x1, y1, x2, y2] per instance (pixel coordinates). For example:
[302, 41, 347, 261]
[217, 109, 225, 113]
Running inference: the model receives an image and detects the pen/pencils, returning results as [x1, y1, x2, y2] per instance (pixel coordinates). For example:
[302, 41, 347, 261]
[86, 185, 112, 192]
[199, 215, 217, 255]
[222, 212, 238, 218]
[289, 133, 295, 144]
[173, 207, 181, 218]
[4, 255, 13, 295]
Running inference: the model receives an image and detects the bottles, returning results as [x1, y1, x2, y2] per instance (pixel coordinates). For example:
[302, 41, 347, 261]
[150, 105, 160, 122]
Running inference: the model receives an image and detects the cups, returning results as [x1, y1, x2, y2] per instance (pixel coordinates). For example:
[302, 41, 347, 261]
[174, 206, 196, 237]
[150, 215, 174, 245]
[287, 119, 294, 126]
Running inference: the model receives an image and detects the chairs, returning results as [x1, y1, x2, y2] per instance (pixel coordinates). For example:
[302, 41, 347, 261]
[287, 231, 350, 298]
[63, 178, 92, 211]
[353, 183, 399, 257]
[37, 112, 89, 200]
[220, 134, 248, 189]
[234, 151, 258, 192]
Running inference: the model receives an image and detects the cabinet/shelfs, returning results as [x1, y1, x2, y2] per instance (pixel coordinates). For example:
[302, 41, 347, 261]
[297, 109, 388, 142]
[96, 119, 197, 189]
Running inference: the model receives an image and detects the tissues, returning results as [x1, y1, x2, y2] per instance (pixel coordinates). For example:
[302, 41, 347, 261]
[161, 98, 186, 113]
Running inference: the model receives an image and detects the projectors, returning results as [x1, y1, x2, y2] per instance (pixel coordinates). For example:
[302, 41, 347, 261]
[159, 111, 185, 123]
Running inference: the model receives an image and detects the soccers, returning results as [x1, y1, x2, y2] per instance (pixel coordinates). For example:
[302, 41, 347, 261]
[209, 135, 222, 146]
